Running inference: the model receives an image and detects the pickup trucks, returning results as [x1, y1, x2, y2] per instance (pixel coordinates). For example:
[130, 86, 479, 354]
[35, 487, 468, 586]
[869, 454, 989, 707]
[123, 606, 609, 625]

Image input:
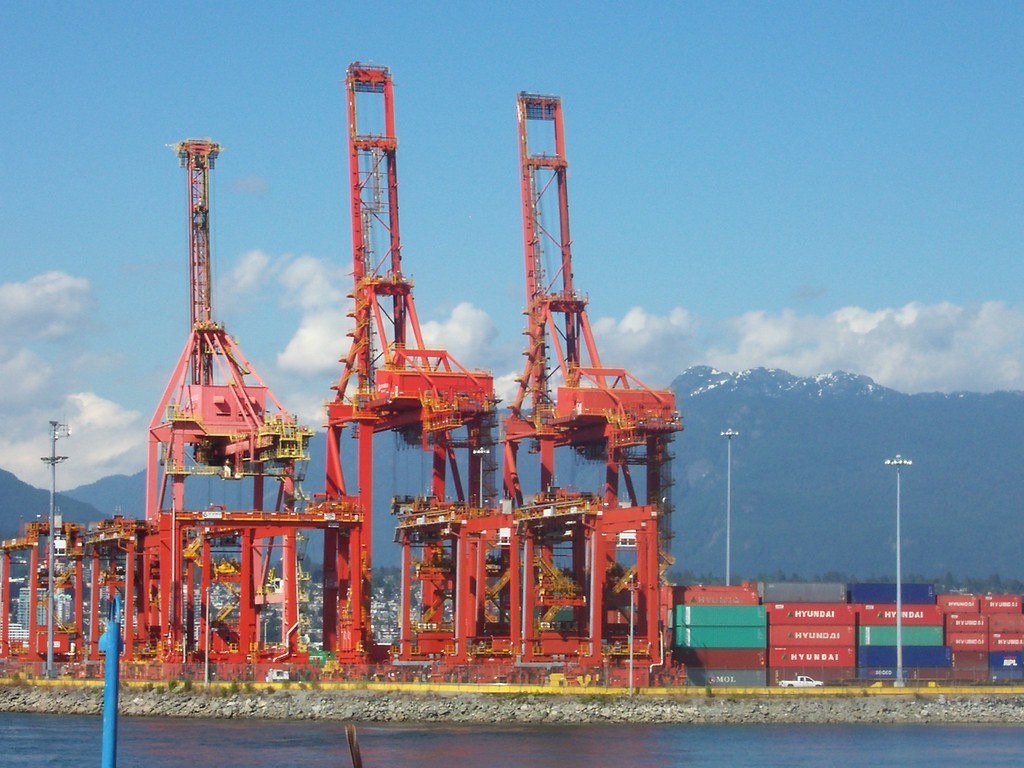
[778, 676, 825, 687]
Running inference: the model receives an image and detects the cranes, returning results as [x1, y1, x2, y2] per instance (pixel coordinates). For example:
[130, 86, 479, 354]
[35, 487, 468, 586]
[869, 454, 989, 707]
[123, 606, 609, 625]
[0, 61, 687, 688]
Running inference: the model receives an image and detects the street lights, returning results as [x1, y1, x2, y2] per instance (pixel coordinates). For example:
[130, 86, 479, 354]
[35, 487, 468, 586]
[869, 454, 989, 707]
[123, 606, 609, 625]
[97, 597, 125, 768]
[721, 428, 739, 586]
[884, 453, 912, 687]
